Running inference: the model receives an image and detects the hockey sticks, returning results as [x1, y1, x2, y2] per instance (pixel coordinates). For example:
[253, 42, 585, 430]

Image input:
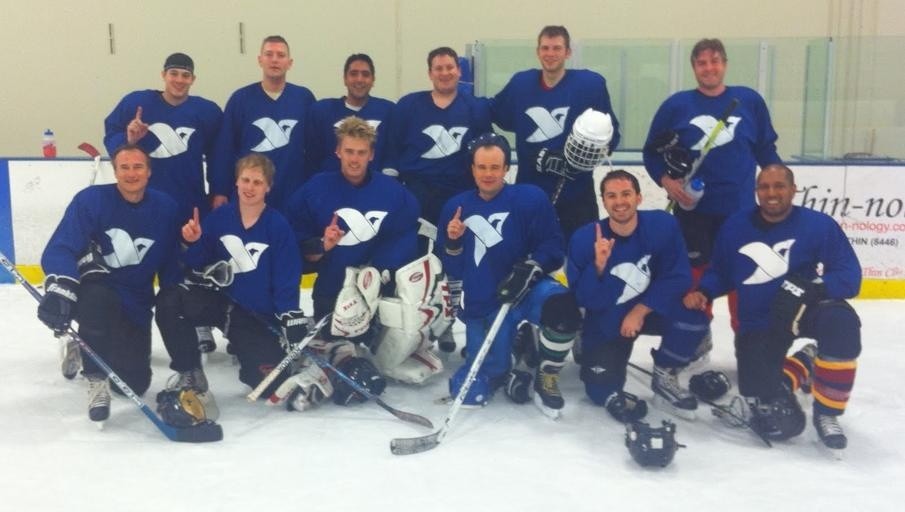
[389, 176, 566, 456]
[216, 286, 436, 429]
[76, 141, 101, 187]
[2, 255, 223, 442]
[631, 362, 773, 450]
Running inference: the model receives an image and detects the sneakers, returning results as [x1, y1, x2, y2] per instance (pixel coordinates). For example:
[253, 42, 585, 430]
[80, 370, 111, 422]
[156, 367, 208, 403]
[196, 326, 216, 353]
[812, 409, 847, 448]
[438, 326, 456, 352]
[61, 340, 81, 379]
[511, 323, 537, 368]
[571, 319, 586, 364]
[533, 356, 567, 409]
[801, 344, 816, 394]
[651, 360, 698, 410]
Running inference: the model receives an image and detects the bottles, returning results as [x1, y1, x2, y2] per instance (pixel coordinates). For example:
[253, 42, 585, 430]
[679, 176, 707, 212]
[40, 128, 56, 158]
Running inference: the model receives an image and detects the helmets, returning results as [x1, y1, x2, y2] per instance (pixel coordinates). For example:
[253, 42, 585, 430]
[749, 394, 805, 442]
[624, 421, 678, 468]
[564, 108, 614, 173]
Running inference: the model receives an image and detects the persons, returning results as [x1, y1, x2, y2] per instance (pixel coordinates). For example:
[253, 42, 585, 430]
[681, 161, 862, 460]
[302, 51, 409, 190]
[437, 130, 582, 419]
[374, 46, 495, 368]
[566, 167, 710, 422]
[643, 38, 786, 371]
[484, 24, 621, 364]
[282, 112, 428, 406]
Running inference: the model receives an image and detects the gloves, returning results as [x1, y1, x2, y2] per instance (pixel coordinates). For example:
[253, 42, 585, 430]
[536, 147, 582, 182]
[496, 261, 544, 310]
[38, 273, 80, 337]
[274, 309, 308, 354]
[647, 127, 695, 180]
[768, 272, 817, 343]
[192, 261, 234, 292]
